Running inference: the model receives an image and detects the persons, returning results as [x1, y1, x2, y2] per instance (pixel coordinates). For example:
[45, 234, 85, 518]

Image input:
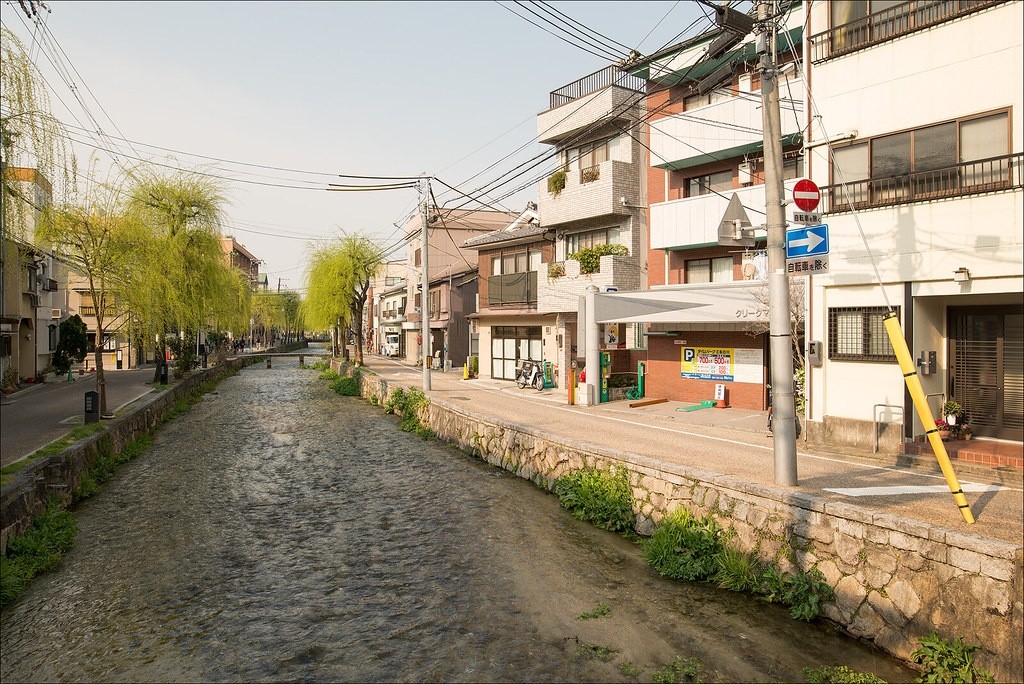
[367, 342, 372, 356]
[256, 338, 261, 350]
[233, 337, 245, 352]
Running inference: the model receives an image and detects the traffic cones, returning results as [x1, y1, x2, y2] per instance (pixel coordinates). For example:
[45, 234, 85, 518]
[67, 369, 73, 381]
[463, 363, 469, 379]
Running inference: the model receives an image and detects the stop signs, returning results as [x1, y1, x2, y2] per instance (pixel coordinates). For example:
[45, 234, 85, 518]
[792, 179, 821, 212]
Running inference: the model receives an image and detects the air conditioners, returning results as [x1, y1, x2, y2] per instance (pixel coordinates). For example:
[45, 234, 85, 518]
[32, 294, 42, 305]
[43, 277, 57, 290]
[52, 308, 61, 319]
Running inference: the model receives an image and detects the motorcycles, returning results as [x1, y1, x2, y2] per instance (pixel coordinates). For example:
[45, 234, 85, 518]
[515, 357, 546, 391]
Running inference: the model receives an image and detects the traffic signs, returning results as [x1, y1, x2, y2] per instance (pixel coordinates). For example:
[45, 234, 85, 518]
[784, 225, 829, 258]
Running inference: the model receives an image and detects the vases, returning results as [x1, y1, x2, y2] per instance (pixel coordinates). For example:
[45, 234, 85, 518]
[938, 430, 952, 441]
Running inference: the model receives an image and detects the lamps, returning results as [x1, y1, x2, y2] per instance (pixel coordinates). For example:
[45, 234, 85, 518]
[26, 333, 32, 340]
[953, 268, 970, 281]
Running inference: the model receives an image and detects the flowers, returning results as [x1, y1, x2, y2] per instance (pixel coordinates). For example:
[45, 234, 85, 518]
[935, 419, 953, 431]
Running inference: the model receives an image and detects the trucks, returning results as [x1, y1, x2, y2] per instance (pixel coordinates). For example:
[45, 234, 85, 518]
[380, 326, 399, 357]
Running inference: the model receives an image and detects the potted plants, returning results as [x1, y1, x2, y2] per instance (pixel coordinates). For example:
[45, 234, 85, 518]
[938, 401, 966, 425]
[42, 365, 57, 382]
[950, 426, 976, 441]
